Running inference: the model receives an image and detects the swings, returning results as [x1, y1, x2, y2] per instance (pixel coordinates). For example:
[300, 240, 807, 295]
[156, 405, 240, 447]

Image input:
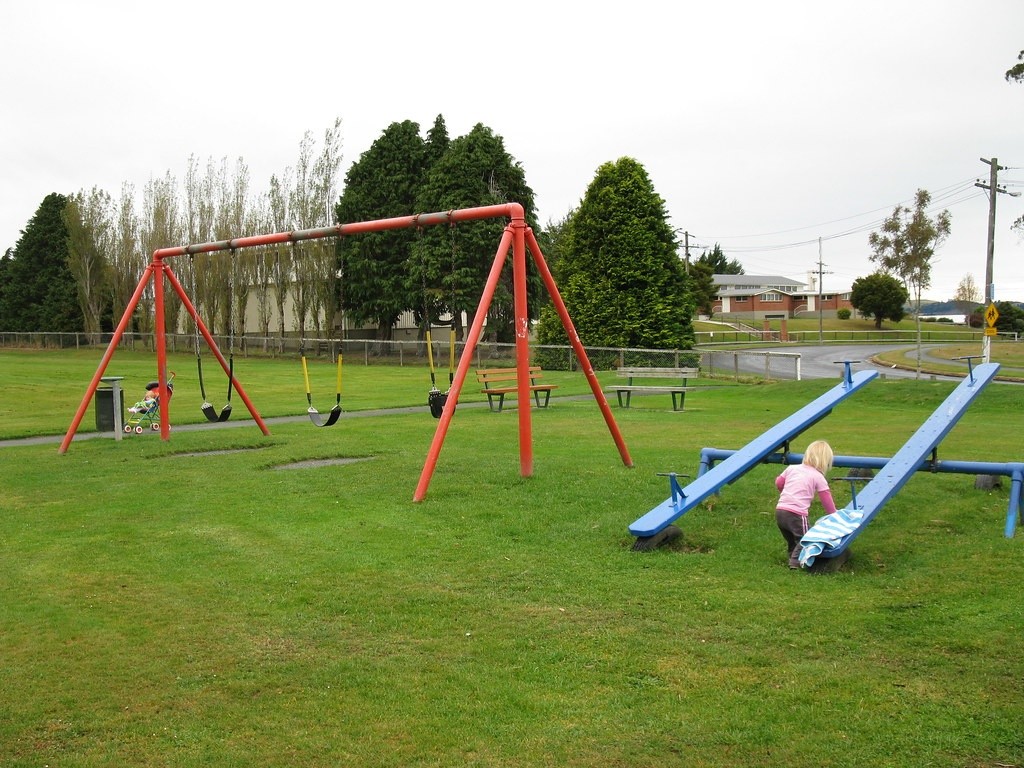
[293, 236, 345, 426]
[191, 255, 235, 423]
[420, 227, 458, 419]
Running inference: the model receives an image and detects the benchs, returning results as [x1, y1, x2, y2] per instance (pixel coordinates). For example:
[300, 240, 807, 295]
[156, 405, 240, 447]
[604, 366, 699, 413]
[476, 367, 558, 413]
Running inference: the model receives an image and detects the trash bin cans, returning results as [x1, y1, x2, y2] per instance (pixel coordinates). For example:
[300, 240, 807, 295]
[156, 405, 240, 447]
[95, 387, 125, 432]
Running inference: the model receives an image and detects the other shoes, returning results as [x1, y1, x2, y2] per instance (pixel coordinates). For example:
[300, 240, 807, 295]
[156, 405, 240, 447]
[790, 566, 798, 570]
[787, 558, 791, 567]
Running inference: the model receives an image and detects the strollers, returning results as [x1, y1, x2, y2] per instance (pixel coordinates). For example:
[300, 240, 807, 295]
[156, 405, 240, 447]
[123, 370, 176, 434]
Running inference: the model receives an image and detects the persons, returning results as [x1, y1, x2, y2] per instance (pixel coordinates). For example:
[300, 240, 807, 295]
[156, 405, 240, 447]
[127, 390, 156, 413]
[775, 440, 838, 570]
